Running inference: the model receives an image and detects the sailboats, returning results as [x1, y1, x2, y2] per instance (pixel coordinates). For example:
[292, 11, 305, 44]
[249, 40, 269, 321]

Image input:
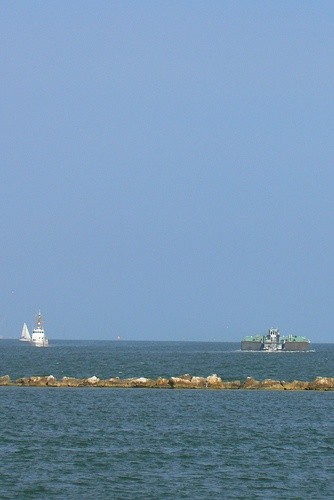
[19, 322, 31, 342]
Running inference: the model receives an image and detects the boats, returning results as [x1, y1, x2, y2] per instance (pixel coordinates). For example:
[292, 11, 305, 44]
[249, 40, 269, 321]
[241, 327, 311, 351]
[31, 312, 48, 347]
